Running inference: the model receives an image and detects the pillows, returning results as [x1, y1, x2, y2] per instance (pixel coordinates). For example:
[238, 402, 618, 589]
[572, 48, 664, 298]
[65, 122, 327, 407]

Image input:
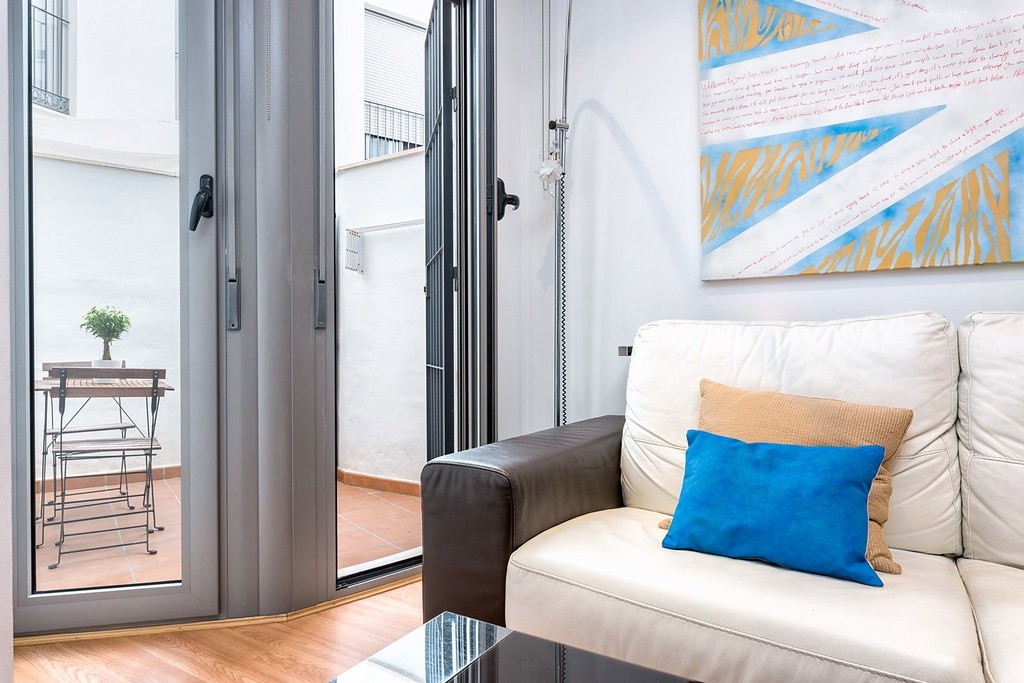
[661, 430, 886, 588]
[658, 380, 914, 578]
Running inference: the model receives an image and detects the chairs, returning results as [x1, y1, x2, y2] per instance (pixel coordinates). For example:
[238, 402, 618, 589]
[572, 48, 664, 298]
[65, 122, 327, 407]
[49, 370, 166, 569]
[42, 362, 137, 520]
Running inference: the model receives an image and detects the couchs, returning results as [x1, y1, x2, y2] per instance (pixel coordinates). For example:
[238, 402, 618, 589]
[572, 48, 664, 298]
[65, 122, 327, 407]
[419, 313, 1024, 683]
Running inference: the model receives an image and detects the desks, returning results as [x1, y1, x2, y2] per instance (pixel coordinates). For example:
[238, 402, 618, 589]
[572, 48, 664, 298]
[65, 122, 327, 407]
[31, 379, 176, 548]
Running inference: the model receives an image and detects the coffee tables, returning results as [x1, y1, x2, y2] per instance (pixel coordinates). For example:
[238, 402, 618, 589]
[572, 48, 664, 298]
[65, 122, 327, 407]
[328, 611, 704, 683]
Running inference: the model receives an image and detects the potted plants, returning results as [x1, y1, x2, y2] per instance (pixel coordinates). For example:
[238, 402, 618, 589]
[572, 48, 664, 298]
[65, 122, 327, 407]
[81, 305, 131, 384]
